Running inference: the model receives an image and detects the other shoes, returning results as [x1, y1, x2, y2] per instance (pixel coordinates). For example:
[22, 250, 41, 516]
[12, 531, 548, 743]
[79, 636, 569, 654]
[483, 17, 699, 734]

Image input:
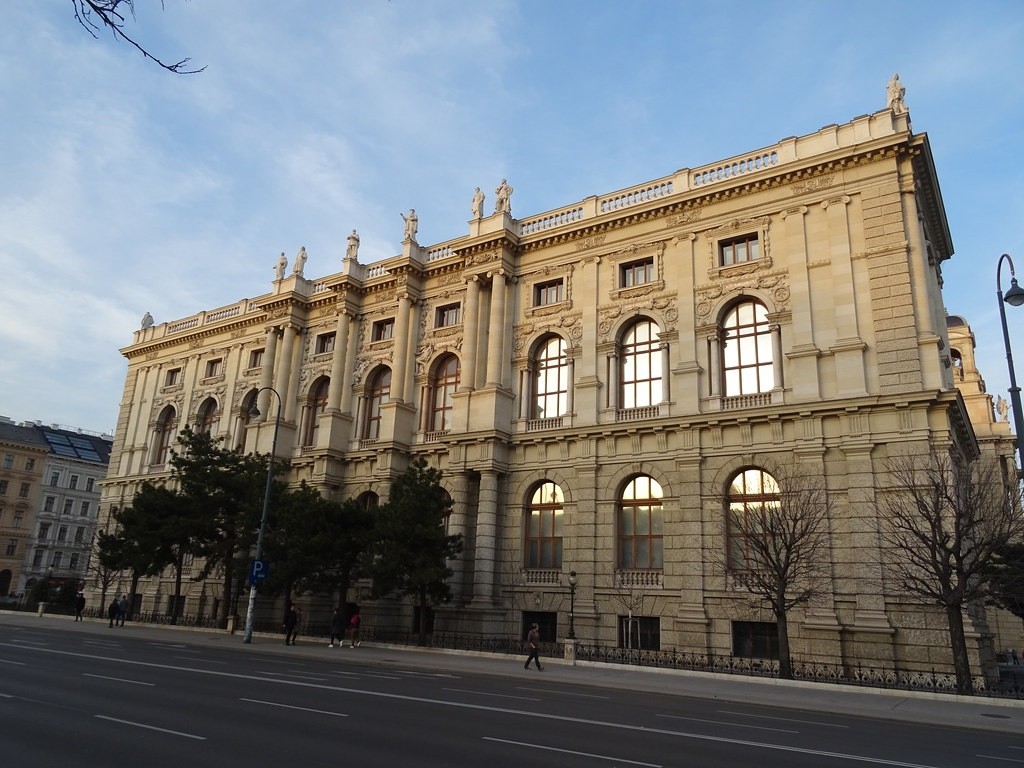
[538, 666, 545, 671]
[328, 644, 333, 647]
[285, 640, 291, 646]
[115, 625, 118, 627]
[74, 620, 77, 622]
[525, 667, 532, 670]
[350, 646, 354, 649]
[108, 626, 113, 628]
[291, 641, 295, 646]
[120, 625, 123, 628]
[339, 641, 343, 647]
[357, 641, 361, 647]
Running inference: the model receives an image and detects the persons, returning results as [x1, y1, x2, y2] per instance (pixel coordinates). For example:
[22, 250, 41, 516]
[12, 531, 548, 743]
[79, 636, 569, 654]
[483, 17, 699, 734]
[73, 593, 85, 622]
[400, 209, 418, 242]
[494, 178, 513, 214]
[141, 312, 154, 330]
[885, 72, 908, 114]
[471, 187, 485, 220]
[327, 609, 361, 649]
[524, 624, 545, 672]
[1011, 648, 1020, 665]
[293, 246, 307, 275]
[273, 252, 288, 280]
[285, 605, 302, 646]
[107, 595, 128, 628]
[343, 229, 360, 261]
[1000, 399, 1012, 421]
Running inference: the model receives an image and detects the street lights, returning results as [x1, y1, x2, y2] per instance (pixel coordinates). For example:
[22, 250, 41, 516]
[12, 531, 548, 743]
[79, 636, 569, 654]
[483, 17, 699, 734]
[41, 562, 56, 598]
[242, 387, 283, 644]
[995, 252, 1024, 470]
[563, 569, 579, 660]
